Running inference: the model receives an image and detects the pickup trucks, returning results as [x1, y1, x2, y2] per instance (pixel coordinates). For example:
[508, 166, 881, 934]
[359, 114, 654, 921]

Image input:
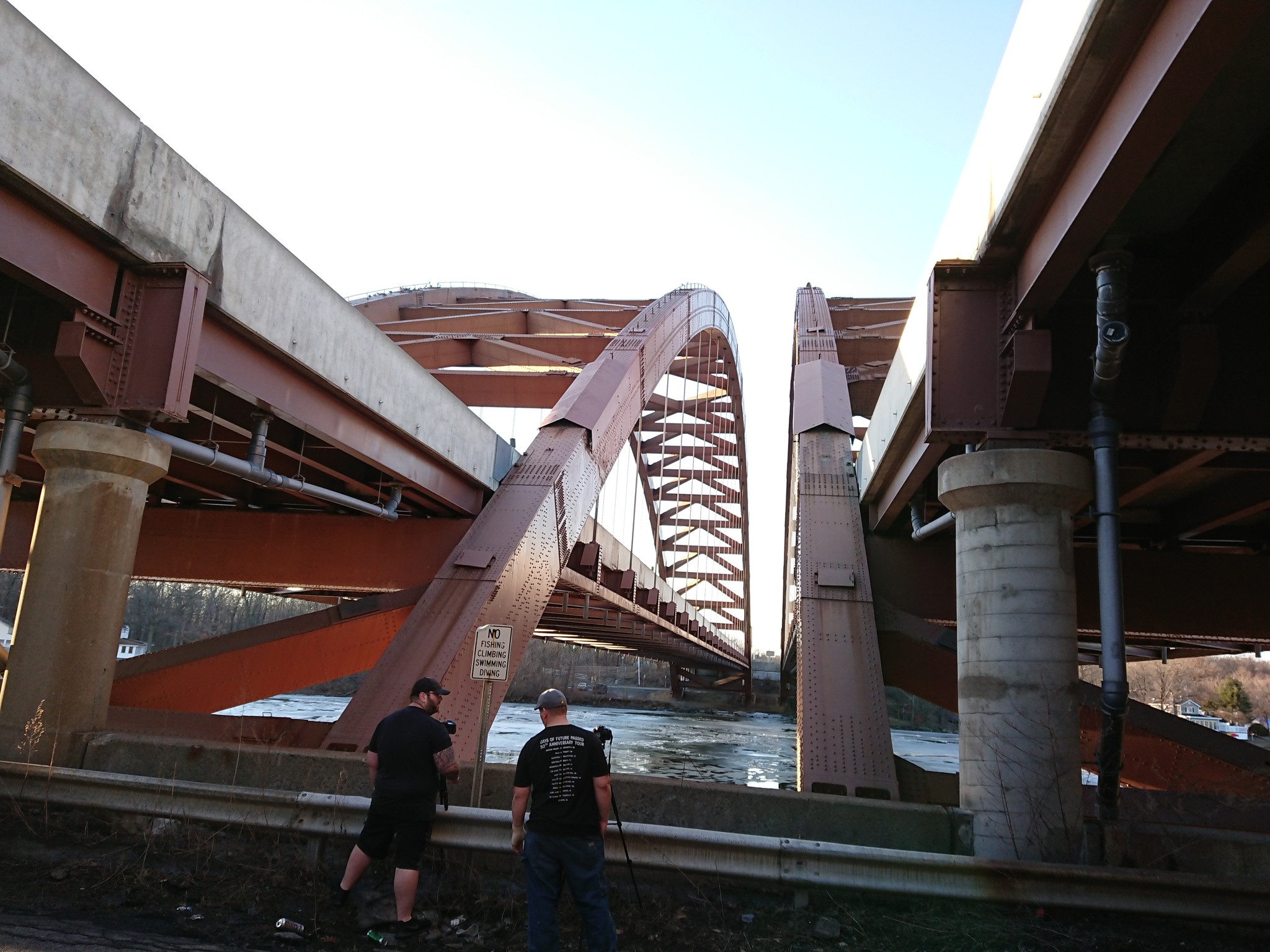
[593, 683, 608, 694]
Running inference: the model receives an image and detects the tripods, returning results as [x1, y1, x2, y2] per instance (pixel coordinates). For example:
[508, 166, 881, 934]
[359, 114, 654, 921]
[557, 746, 644, 952]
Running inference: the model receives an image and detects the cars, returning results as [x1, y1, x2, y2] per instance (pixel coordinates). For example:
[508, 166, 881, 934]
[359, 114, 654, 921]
[577, 683, 588, 690]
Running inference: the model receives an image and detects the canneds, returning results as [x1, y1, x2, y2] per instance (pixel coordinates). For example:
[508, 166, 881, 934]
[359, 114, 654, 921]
[276, 918, 304, 936]
[176, 906, 195, 913]
[190, 915, 202, 919]
[366, 930, 387, 946]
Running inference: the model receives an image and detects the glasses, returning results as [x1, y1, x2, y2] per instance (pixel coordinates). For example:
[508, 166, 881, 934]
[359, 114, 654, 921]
[429, 692, 439, 696]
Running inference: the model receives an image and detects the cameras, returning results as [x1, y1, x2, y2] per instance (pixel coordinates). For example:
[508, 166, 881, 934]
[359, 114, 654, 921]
[592, 725, 611, 744]
[442, 720, 457, 734]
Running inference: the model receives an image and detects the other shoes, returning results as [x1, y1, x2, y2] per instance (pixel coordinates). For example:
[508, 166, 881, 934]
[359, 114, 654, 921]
[331, 880, 345, 907]
[394, 917, 432, 940]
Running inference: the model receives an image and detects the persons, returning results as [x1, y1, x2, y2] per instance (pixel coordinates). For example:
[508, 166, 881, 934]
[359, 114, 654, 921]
[510, 690, 611, 952]
[331, 676, 460, 941]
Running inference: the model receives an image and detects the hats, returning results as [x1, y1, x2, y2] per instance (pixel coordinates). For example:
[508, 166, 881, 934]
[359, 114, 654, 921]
[410, 677, 451, 696]
[534, 688, 567, 710]
